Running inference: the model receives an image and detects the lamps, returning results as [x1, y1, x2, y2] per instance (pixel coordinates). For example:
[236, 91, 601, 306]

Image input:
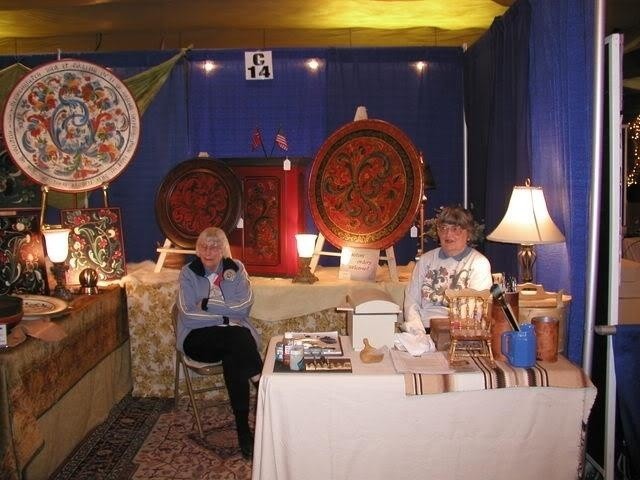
[41, 229, 71, 302]
[292, 233, 319, 284]
[485, 177, 566, 289]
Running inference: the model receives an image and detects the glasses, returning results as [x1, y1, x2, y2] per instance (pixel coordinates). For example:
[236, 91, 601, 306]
[437, 224, 468, 238]
[197, 243, 223, 252]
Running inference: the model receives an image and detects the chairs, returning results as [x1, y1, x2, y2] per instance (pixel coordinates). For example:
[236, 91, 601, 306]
[442, 289, 496, 367]
[171, 302, 258, 440]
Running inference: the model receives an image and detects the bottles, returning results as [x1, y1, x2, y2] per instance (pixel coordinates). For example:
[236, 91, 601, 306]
[290, 340, 304, 371]
[275, 342, 283, 362]
[283, 331, 293, 367]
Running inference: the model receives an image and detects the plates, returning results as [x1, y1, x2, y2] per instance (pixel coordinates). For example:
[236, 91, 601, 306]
[13, 294, 67, 320]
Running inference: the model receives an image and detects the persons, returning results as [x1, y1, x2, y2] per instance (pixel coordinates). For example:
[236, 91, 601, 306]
[403, 206, 493, 334]
[175, 227, 264, 458]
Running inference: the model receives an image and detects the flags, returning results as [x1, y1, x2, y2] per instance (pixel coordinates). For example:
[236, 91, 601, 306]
[273, 130, 289, 152]
[247, 127, 263, 152]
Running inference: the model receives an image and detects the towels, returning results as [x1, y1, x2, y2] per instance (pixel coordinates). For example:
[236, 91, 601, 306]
[395, 328, 435, 357]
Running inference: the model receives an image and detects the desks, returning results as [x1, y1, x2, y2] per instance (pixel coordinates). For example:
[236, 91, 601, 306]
[251, 332, 598, 480]
[185, 156, 312, 278]
[122, 265, 415, 399]
[0, 274, 134, 480]
